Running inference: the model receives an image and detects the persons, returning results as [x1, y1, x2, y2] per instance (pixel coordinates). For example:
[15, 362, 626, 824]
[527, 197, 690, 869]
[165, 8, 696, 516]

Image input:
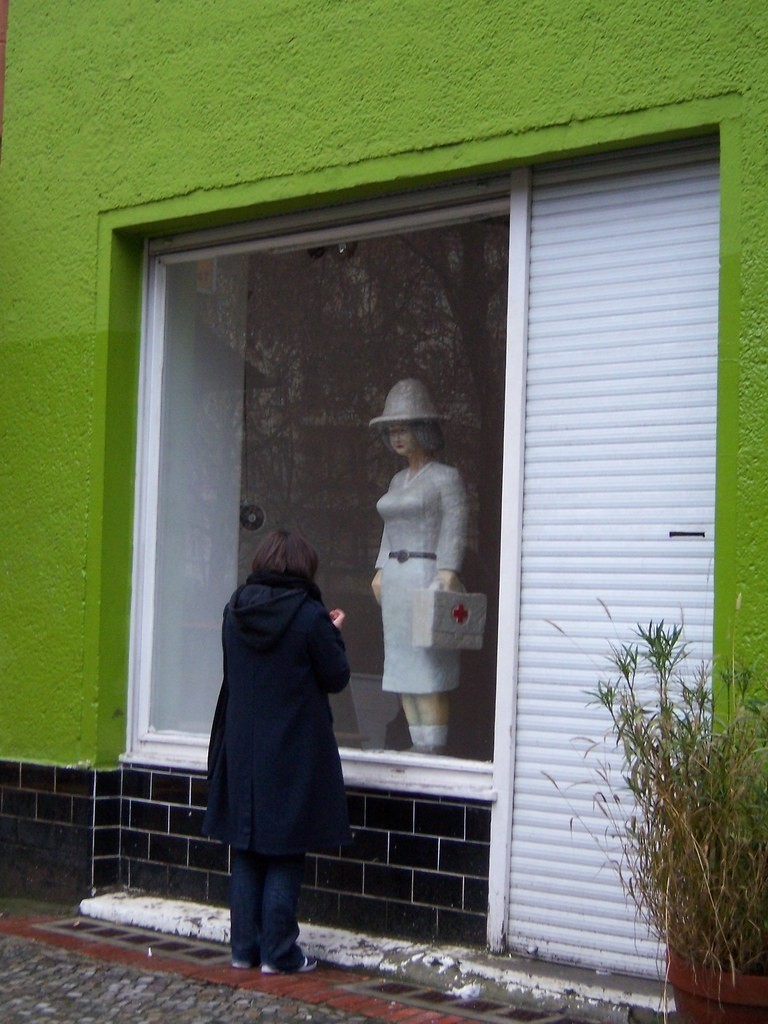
[202, 529, 352, 975]
[368, 378, 470, 755]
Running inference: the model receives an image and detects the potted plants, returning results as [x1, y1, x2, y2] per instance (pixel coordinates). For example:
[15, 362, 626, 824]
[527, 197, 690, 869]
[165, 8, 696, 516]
[530, 582, 768, 1024]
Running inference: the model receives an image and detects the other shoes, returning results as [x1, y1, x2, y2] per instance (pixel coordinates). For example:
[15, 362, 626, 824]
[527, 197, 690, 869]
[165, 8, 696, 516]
[231, 954, 261, 968]
[261, 956, 318, 972]
